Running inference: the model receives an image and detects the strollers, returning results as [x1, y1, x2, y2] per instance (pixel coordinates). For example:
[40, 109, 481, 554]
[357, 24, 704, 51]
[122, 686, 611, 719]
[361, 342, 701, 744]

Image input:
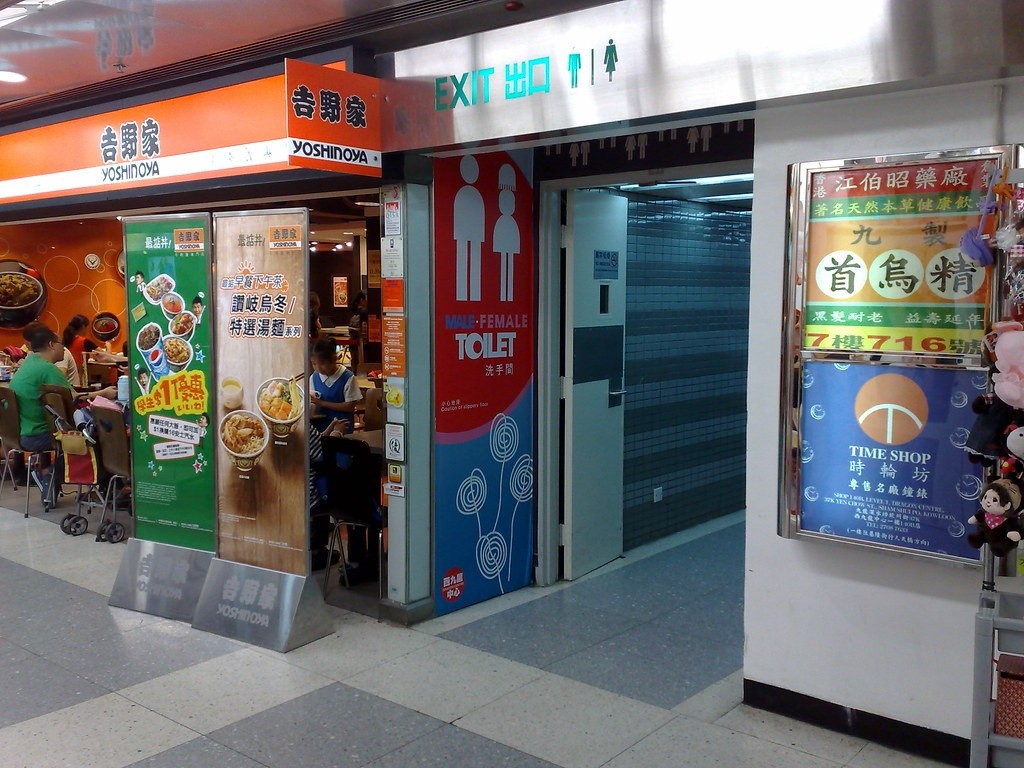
[43, 393, 133, 544]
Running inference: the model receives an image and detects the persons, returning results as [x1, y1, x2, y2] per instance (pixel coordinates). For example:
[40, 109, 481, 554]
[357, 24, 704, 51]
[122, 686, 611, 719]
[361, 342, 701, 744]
[308, 291, 388, 569]
[8, 314, 130, 512]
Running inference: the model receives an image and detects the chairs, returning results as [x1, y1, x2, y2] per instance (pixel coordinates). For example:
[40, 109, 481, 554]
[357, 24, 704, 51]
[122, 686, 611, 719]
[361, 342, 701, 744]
[86, 364, 110, 384]
[310, 436, 383, 600]
[0, 384, 131, 542]
[357, 363, 382, 376]
[365, 388, 383, 432]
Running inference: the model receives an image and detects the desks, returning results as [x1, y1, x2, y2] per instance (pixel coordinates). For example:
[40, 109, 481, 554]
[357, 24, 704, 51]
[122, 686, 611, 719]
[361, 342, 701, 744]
[329, 337, 365, 364]
[354, 375, 376, 389]
[344, 430, 383, 454]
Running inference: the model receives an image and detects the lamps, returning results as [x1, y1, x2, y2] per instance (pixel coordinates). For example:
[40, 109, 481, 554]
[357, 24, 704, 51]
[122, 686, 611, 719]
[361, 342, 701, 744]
[355, 194, 384, 208]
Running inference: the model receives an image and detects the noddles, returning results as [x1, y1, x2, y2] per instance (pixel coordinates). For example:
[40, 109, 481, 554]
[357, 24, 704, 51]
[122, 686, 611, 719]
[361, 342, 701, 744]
[0, 273, 39, 307]
[259, 376, 304, 421]
[222, 414, 265, 455]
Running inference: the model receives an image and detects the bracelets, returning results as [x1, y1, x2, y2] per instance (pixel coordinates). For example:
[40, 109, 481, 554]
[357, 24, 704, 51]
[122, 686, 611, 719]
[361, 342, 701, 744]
[330, 430, 343, 438]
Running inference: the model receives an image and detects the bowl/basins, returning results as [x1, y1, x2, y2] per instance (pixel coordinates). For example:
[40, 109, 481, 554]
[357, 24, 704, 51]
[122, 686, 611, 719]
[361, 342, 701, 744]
[219, 410, 269, 470]
[94, 318, 118, 337]
[162, 335, 193, 373]
[168, 311, 197, 341]
[258, 378, 306, 438]
[161, 293, 185, 321]
[0, 271, 44, 321]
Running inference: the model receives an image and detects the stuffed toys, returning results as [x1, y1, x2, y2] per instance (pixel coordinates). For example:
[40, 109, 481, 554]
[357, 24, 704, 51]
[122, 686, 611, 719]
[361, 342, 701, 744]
[966, 332, 1024, 557]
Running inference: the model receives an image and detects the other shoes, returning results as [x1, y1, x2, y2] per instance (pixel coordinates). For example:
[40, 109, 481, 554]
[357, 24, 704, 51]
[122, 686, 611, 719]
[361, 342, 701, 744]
[41, 473, 58, 511]
[312, 549, 340, 570]
[339, 566, 369, 587]
[111, 483, 132, 507]
[76, 489, 105, 502]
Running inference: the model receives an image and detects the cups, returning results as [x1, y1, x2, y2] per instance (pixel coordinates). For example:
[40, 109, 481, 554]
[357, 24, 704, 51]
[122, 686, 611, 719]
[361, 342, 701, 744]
[221, 377, 244, 409]
[0, 366, 10, 380]
[136, 323, 169, 382]
[117, 376, 129, 401]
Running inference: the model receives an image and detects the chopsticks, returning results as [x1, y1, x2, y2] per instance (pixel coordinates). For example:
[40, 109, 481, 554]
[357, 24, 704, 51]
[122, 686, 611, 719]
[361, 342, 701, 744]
[289, 371, 305, 384]
